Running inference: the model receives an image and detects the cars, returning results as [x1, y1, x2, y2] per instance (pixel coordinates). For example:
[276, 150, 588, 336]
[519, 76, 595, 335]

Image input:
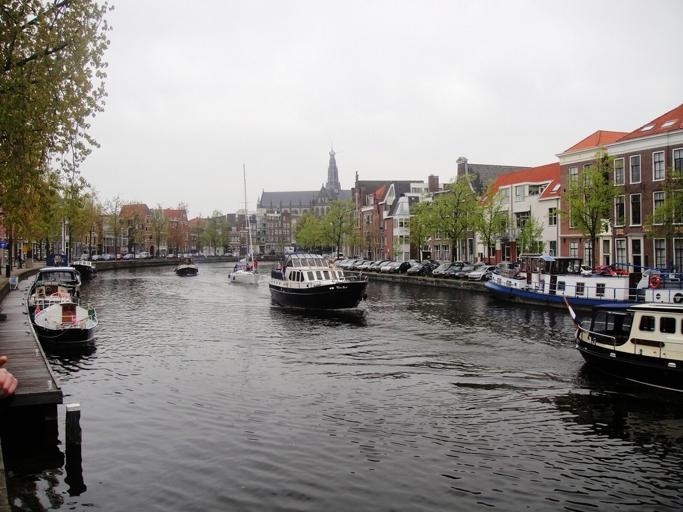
[80, 252, 151, 261]
[322, 251, 517, 282]
[166, 254, 188, 258]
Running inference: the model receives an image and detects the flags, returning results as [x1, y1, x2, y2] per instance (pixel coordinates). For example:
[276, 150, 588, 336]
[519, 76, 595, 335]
[564, 299, 581, 327]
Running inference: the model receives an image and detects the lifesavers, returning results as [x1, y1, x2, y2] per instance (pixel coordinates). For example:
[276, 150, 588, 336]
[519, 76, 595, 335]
[650, 276, 660, 289]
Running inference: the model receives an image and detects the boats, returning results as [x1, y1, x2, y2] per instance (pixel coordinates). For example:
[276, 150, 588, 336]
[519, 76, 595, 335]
[27, 259, 100, 349]
[176, 257, 198, 275]
[268, 252, 368, 309]
[228, 257, 260, 284]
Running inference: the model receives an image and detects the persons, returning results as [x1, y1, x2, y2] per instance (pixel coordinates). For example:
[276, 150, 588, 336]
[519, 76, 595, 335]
[233, 256, 258, 274]
[0, 353, 21, 401]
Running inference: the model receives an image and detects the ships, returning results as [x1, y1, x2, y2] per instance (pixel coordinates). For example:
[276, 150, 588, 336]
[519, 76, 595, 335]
[484, 254, 683, 316]
[574, 302, 683, 403]
